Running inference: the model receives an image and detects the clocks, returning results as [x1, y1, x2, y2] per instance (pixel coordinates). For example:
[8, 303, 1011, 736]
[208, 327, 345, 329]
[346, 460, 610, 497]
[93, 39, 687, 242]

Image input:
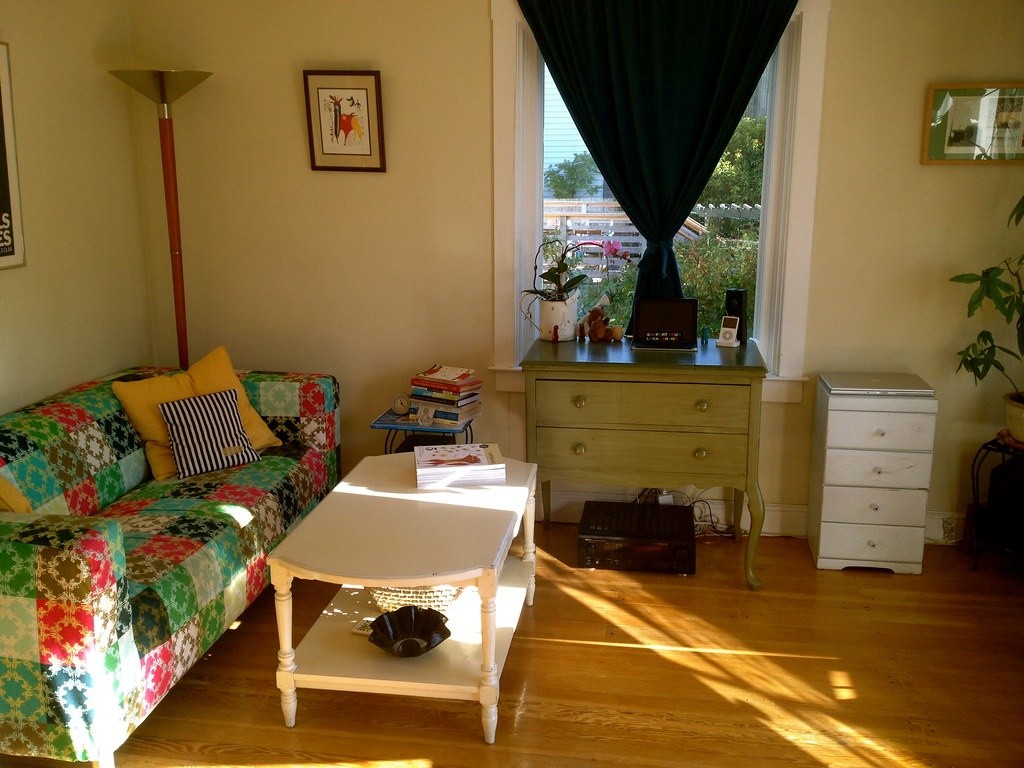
[390, 393, 411, 414]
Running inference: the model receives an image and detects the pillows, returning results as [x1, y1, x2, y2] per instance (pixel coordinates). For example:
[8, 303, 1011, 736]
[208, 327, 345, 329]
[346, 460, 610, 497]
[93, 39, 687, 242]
[156, 389, 263, 480]
[111, 345, 283, 481]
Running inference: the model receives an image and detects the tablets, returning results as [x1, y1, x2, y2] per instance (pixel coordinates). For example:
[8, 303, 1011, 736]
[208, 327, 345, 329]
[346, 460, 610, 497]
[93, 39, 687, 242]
[632, 298, 698, 351]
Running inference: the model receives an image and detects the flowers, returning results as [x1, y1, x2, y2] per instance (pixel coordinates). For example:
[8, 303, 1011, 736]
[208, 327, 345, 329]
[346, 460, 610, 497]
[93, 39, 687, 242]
[519, 238, 631, 331]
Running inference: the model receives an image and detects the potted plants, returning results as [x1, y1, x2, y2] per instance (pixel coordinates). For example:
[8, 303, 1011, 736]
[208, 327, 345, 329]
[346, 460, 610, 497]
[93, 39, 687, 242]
[948, 195, 1024, 444]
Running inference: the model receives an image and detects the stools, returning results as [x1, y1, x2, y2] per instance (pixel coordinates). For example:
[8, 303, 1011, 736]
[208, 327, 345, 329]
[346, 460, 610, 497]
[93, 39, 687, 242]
[971, 435, 1024, 509]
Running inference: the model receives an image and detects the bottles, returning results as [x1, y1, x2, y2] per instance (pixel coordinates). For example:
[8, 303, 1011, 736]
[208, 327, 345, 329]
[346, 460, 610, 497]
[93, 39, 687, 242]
[700, 324, 710, 346]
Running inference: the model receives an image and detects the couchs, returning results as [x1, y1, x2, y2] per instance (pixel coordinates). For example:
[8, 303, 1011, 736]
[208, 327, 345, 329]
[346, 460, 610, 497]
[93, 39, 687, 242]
[0, 344, 343, 768]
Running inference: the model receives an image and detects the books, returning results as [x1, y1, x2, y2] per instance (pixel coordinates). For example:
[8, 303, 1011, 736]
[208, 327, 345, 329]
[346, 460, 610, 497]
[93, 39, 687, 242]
[409, 364, 483, 424]
[414, 442, 506, 487]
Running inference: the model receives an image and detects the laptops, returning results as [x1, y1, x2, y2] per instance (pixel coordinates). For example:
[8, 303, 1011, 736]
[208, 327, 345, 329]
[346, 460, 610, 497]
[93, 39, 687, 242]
[818, 372, 935, 398]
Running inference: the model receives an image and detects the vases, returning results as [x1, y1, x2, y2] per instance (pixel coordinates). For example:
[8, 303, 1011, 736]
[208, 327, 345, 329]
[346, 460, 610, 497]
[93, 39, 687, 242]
[539, 299, 576, 341]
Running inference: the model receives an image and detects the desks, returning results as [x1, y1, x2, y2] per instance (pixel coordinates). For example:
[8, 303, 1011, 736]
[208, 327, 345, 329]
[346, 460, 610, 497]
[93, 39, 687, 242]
[518, 335, 769, 589]
[369, 401, 485, 455]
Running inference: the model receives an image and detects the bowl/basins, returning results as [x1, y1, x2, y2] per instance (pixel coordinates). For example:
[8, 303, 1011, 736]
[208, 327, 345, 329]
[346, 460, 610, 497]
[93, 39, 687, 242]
[368, 605, 451, 658]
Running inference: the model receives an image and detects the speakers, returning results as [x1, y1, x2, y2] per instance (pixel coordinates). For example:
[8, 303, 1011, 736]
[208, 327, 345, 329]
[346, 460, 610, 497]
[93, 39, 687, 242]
[725, 288, 748, 344]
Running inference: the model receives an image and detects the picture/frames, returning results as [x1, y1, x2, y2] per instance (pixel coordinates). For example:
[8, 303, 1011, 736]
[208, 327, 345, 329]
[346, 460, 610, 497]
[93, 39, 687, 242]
[303, 70, 386, 173]
[920, 81, 1024, 166]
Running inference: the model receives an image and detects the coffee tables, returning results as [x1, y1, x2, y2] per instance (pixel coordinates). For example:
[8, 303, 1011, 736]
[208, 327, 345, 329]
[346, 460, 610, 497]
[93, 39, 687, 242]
[267, 451, 537, 745]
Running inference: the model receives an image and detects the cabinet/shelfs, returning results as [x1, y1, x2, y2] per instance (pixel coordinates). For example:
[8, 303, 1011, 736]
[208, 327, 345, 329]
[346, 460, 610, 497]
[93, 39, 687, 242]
[806, 373, 939, 575]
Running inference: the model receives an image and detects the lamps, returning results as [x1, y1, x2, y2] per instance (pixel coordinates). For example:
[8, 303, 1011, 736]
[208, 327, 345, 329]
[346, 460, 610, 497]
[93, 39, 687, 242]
[107, 67, 214, 368]
